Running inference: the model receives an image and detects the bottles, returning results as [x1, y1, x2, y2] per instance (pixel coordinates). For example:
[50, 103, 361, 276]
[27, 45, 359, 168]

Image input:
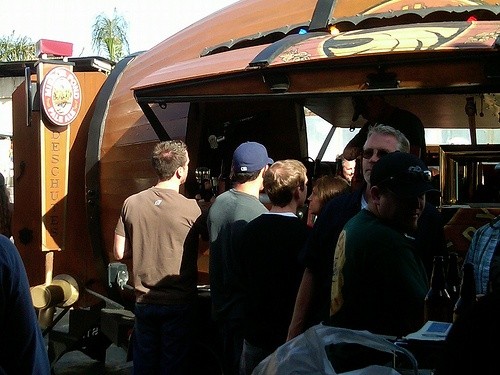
[446, 252, 459, 313]
[423, 256, 450, 324]
[453, 262, 476, 324]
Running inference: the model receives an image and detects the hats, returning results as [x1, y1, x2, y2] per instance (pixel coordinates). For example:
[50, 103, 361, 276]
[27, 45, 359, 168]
[369, 151, 434, 195]
[230, 141, 274, 172]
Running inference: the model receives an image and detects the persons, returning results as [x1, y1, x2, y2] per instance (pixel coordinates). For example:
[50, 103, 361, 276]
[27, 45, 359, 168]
[112, 96, 448, 375]
[0, 172, 51, 375]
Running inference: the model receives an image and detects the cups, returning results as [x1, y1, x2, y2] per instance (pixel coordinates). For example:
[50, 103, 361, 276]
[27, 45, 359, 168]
[116, 271, 129, 291]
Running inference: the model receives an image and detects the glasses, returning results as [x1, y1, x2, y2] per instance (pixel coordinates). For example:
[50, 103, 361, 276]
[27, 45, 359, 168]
[363, 148, 391, 159]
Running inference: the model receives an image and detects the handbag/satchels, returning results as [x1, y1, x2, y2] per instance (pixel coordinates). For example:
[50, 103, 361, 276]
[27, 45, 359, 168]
[251, 322, 418, 375]
